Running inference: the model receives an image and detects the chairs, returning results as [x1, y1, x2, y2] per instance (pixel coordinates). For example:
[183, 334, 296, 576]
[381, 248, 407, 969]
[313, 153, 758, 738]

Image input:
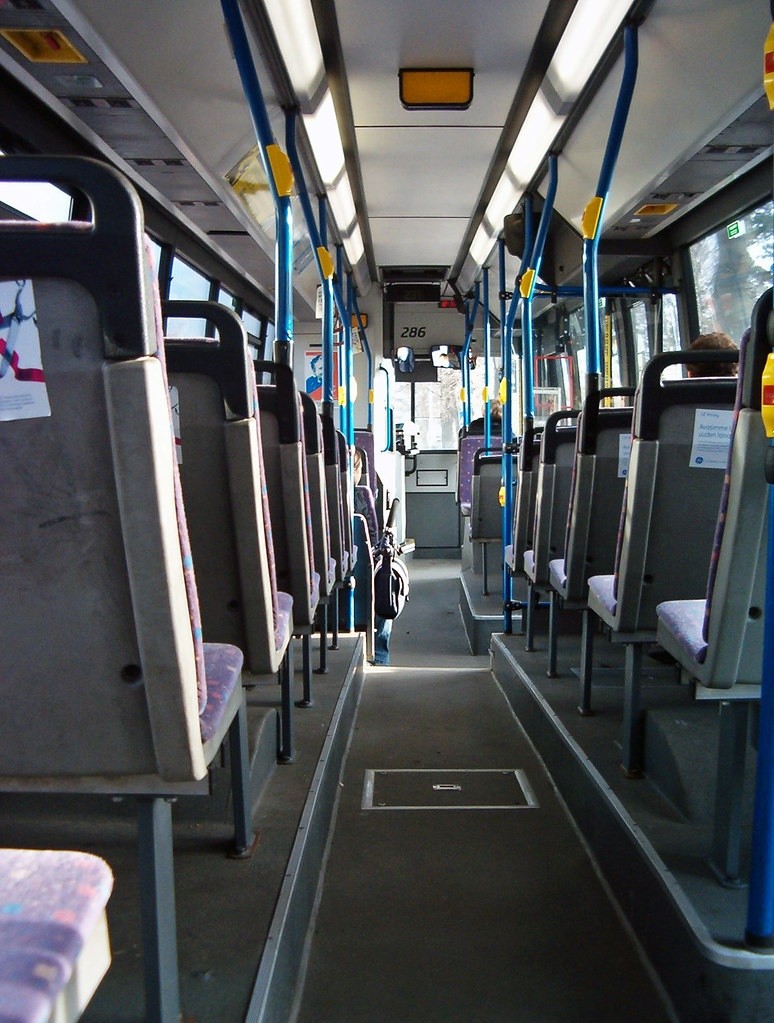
[0, 152, 416, 1021]
[457, 286, 773, 893]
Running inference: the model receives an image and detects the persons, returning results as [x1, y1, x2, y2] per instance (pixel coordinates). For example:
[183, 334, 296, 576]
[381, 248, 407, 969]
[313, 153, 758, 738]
[468, 399, 502, 435]
[685, 332, 739, 378]
[353, 449, 378, 547]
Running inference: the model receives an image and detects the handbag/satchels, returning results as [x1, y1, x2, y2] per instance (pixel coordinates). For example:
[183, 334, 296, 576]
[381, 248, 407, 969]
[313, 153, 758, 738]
[373, 552, 410, 620]
